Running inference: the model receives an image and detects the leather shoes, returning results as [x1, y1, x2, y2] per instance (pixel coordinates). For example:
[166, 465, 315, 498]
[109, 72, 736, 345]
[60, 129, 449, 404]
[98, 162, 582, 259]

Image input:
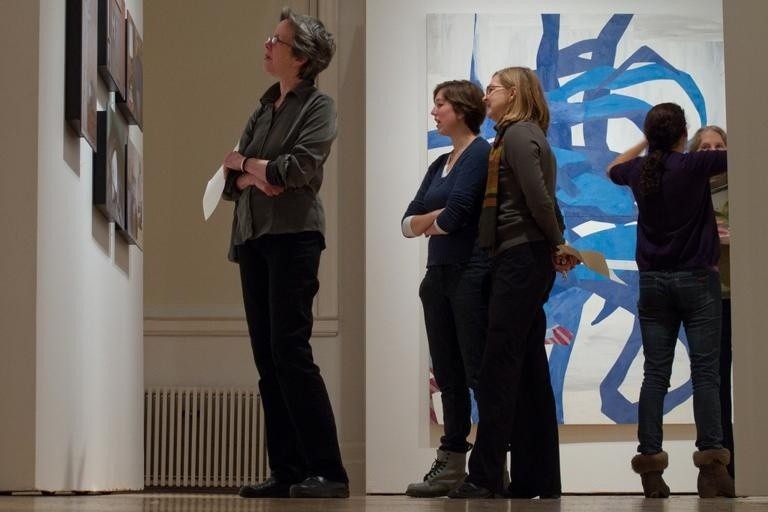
[238, 477, 291, 498]
[448, 482, 501, 499]
[290, 476, 350, 498]
[493, 489, 562, 499]
[405, 449, 467, 498]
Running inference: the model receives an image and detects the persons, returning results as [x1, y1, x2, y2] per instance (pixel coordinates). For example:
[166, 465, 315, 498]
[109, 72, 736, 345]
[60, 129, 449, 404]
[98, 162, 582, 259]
[401, 80, 510, 498]
[220, 5, 350, 499]
[448, 66, 582, 499]
[687, 123, 734, 498]
[605, 104, 732, 498]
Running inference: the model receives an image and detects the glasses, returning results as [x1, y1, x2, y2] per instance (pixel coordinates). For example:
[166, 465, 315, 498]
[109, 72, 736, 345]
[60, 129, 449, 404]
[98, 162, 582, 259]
[267, 36, 295, 49]
[486, 85, 504, 95]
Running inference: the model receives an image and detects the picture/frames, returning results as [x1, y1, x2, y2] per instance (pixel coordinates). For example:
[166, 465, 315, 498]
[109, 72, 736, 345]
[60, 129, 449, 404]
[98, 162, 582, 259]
[116, 8, 144, 132]
[66, 2, 98, 156]
[94, 109, 126, 234]
[97, 1, 126, 104]
[117, 111, 143, 254]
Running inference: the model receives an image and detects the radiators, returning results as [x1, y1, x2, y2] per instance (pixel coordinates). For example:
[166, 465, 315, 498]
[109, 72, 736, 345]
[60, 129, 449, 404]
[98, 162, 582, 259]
[145, 387, 271, 488]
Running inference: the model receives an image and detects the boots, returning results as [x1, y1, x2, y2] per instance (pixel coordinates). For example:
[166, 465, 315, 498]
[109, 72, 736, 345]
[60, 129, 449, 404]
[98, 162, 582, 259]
[631, 451, 670, 498]
[692, 448, 734, 499]
